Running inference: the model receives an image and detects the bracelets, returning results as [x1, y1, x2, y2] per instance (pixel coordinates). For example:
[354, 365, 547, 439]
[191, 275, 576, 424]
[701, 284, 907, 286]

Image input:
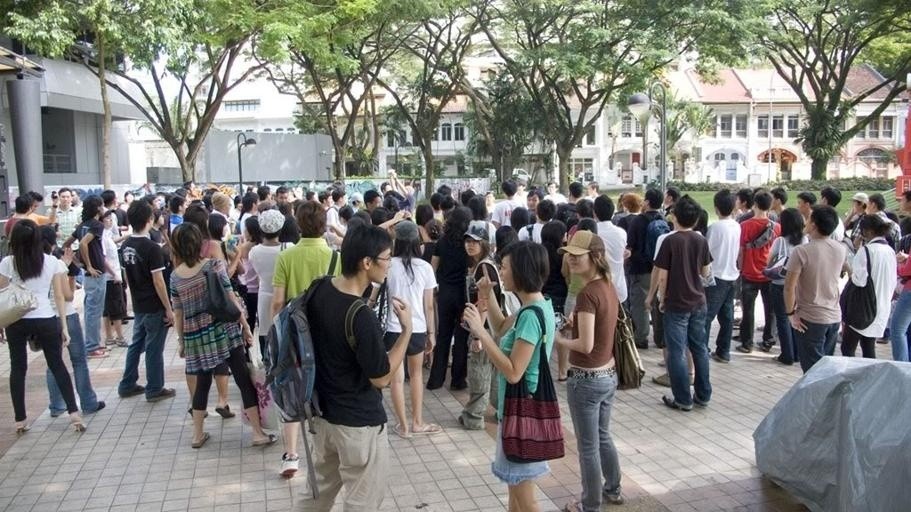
[472, 335, 480, 340]
[786, 310, 796, 316]
[177, 334, 184, 345]
[368, 297, 375, 303]
[52, 205, 58, 209]
[427, 331, 434, 335]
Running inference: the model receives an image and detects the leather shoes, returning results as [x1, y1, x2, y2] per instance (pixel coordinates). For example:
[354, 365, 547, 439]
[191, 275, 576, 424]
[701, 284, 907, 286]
[118, 386, 144, 396]
[97, 400, 103, 409]
[145, 388, 174, 401]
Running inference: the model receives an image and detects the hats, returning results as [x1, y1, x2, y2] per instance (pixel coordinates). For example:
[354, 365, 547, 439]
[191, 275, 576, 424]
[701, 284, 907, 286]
[393, 220, 418, 241]
[258, 209, 285, 234]
[347, 192, 364, 202]
[847, 193, 868, 204]
[100, 206, 116, 219]
[464, 225, 488, 241]
[556, 230, 605, 255]
[211, 191, 228, 210]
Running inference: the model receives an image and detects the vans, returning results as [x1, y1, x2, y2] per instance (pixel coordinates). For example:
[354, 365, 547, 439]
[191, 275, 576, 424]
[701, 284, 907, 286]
[486, 168, 533, 182]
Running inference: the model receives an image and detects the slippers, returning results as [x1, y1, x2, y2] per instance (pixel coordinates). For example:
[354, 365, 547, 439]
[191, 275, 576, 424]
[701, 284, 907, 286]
[412, 424, 441, 434]
[393, 423, 412, 438]
[215, 405, 233, 417]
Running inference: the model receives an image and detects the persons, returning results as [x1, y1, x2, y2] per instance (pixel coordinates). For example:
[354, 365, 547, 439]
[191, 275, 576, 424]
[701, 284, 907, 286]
[731, 189, 755, 330]
[556, 203, 579, 229]
[556, 230, 624, 512]
[47, 188, 83, 290]
[150, 210, 168, 247]
[784, 205, 849, 374]
[738, 192, 783, 353]
[843, 193, 869, 234]
[645, 196, 715, 412]
[248, 209, 297, 361]
[625, 190, 672, 349]
[173, 205, 250, 419]
[706, 189, 742, 363]
[899, 190, 911, 236]
[239, 215, 264, 362]
[559, 218, 598, 322]
[769, 188, 788, 215]
[694, 209, 709, 236]
[101, 180, 585, 202]
[796, 192, 818, 228]
[272, 202, 345, 477]
[279, 215, 300, 244]
[119, 199, 176, 403]
[496, 225, 520, 251]
[318, 202, 427, 244]
[427, 206, 475, 391]
[512, 207, 528, 233]
[171, 198, 186, 223]
[851, 194, 902, 250]
[38, 225, 106, 417]
[419, 218, 443, 263]
[362, 221, 443, 437]
[518, 200, 556, 243]
[187, 202, 304, 218]
[462, 241, 558, 512]
[23, 191, 60, 225]
[643, 223, 706, 387]
[460, 223, 506, 430]
[475, 202, 516, 225]
[63, 195, 111, 358]
[889, 242, 911, 362]
[99, 205, 134, 347]
[0, 219, 88, 433]
[840, 214, 898, 358]
[820, 188, 846, 242]
[209, 213, 246, 279]
[4, 193, 35, 240]
[593, 194, 631, 313]
[169, 223, 278, 449]
[767, 207, 810, 365]
[664, 187, 681, 216]
[541, 221, 574, 381]
[565, 199, 595, 245]
[584, 180, 600, 202]
[69, 189, 83, 211]
[296, 224, 415, 511]
[612, 192, 628, 226]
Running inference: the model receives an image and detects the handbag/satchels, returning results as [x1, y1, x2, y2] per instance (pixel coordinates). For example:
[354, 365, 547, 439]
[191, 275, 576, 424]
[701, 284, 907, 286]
[840, 277, 876, 329]
[0, 285, 38, 327]
[500, 288, 521, 318]
[762, 254, 788, 280]
[0, 234, 14, 257]
[204, 269, 240, 323]
[613, 310, 645, 389]
[751, 226, 772, 247]
[503, 361, 565, 463]
[369, 280, 389, 339]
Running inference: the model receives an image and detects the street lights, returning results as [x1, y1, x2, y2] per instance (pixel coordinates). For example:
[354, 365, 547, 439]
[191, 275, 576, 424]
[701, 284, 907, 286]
[627, 81, 669, 206]
[236, 132, 258, 201]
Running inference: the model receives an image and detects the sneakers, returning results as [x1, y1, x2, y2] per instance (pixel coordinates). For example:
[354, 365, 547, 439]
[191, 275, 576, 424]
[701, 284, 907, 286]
[692, 395, 708, 407]
[105, 338, 116, 345]
[735, 345, 751, 352]
[662, 396, 694, 411]
[565, 501, 591, 511]
[279, 451, 299, 478]
[710, 351, 728, 363]
[86, 350, 109, 357]
[757, 341, 771, 352]
[604, 490, 622, 503]
[116, 337, 132, 347]
[99, 345, 111, 351]
[652, 374, 672, 386]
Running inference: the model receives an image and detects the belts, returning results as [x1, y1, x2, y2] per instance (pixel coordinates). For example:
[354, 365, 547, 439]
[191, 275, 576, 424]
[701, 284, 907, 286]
[566, 366, 615, 378]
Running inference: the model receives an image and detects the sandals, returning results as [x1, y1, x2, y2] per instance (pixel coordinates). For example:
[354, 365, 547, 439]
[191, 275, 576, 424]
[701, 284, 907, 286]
[253, 434, 277, 445]
[191, 430, 210, 447]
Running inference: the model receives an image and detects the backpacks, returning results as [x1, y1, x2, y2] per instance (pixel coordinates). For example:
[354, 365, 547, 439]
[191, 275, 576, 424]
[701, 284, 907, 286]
[262, 276, 325, 422]
[641, 213, 671, 266]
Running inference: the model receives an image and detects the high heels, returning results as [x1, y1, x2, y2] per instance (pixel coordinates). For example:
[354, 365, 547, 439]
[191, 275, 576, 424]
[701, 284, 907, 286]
[16, 425, 30, 435]
[74, 422, 87, 431]
